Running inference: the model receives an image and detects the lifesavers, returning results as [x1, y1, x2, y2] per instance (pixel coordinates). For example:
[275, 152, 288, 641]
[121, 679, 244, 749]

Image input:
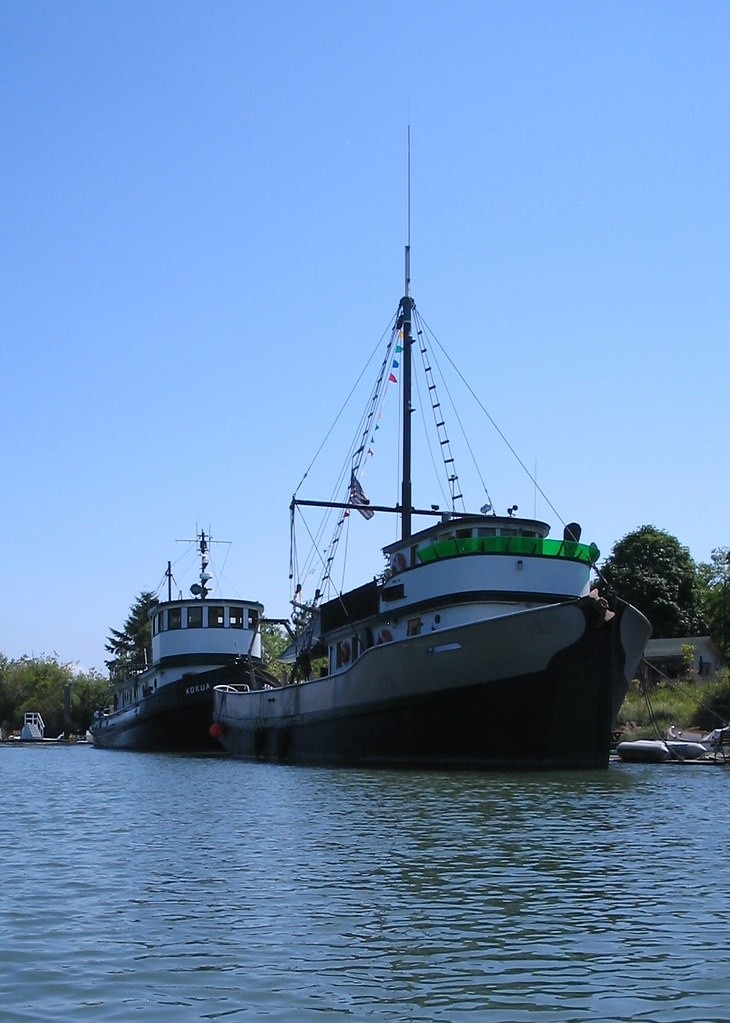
[391, 552, 406, 575]
[339, 642, 349, 662]
[376, 629, 393, 645]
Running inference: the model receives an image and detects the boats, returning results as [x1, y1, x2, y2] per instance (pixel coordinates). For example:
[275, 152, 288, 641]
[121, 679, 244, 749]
[18, 711, 45, 741]
[615, 725, 730, 763]
[84, 527, 284, 753]
[210, 123, 655, 769]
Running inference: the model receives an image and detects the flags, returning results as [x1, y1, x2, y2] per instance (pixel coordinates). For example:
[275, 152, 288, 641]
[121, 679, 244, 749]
[395, 346, 404, 353]
[392, 360, 399, 368]
[399, 332, 404, 339]
[351, 474, 374, 521]
[389, 373, 397, 383]
[368, 449, 374, 456]
